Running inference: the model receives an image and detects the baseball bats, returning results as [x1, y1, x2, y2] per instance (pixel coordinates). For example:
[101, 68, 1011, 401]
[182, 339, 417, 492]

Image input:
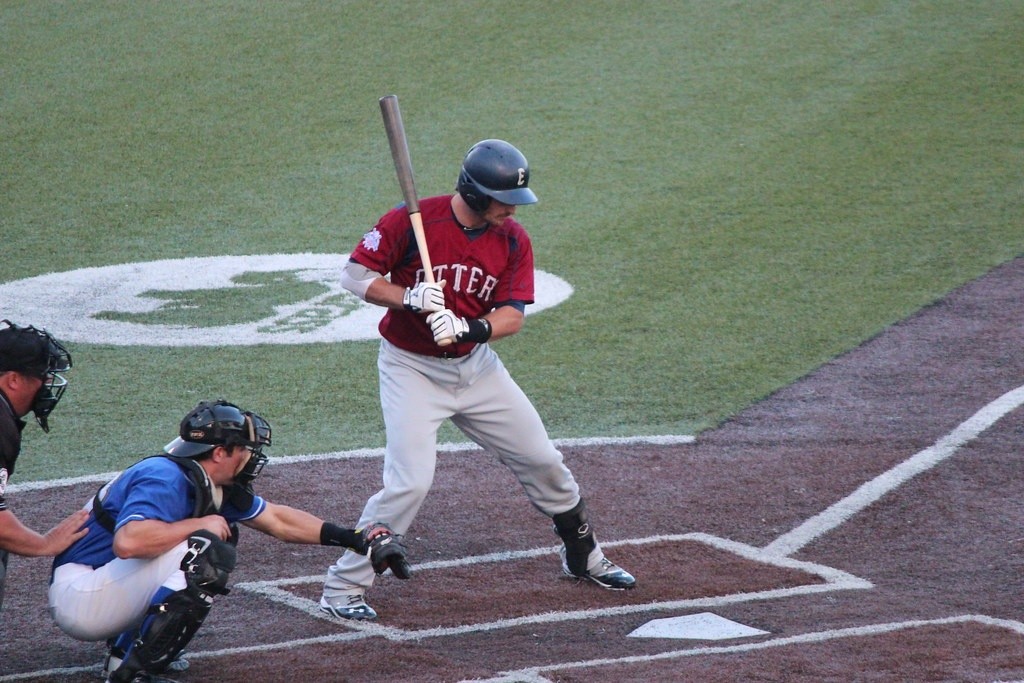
[380, 94, 452, 346]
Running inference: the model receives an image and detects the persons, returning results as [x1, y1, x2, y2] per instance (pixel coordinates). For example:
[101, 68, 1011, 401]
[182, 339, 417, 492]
[48, 399, 412, 683]
[0, 329, 90, 610]
[319, 138, 635, 621]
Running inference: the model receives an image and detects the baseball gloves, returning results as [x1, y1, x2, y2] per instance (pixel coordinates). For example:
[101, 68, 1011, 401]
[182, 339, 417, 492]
[364, 521, 411, 580]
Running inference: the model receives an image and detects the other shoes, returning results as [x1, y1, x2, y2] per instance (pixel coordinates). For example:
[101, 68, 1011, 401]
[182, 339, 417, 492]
[101, 654, 190, 679]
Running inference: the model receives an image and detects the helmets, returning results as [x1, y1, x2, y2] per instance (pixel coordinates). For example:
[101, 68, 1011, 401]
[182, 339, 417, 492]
[163, 399, 250, 458]
[457, 138, 538, 211]
[0, 326, 64, 371]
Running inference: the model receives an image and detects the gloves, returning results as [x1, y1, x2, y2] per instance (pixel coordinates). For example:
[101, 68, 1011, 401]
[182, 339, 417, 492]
[426, 306, 493, 345]
[403, 282, 444, 314]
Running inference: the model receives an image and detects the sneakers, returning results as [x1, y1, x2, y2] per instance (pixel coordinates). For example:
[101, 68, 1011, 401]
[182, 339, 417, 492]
[559, 545, 636, 590]
[319, 596, 377, 621]
